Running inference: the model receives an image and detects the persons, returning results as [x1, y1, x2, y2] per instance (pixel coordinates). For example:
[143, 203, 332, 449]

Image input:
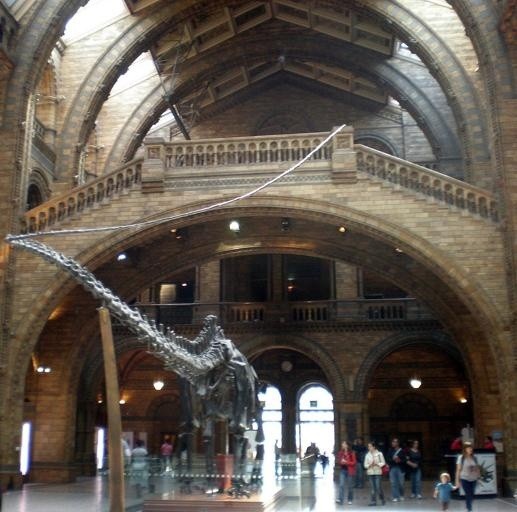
[131, 440, 148, 496]
[303, 434, 480, 511]
[159, 439, 173, 471]
[482, 435, 495, 448]
[273, 439, 281, 476]
[120, 436, 131, 493]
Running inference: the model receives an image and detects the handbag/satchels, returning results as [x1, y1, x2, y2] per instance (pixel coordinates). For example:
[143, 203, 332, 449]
[382, 464, 389, 475]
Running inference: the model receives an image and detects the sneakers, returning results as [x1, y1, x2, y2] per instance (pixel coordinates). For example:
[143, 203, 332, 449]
[368, 503, 376, 506]
[382, 500, 385, 505]
[348, 501, 352, 505]
[392, 496, 405, 502]
[336, 500, 340, 503]
[410, 494, 422, 500]
